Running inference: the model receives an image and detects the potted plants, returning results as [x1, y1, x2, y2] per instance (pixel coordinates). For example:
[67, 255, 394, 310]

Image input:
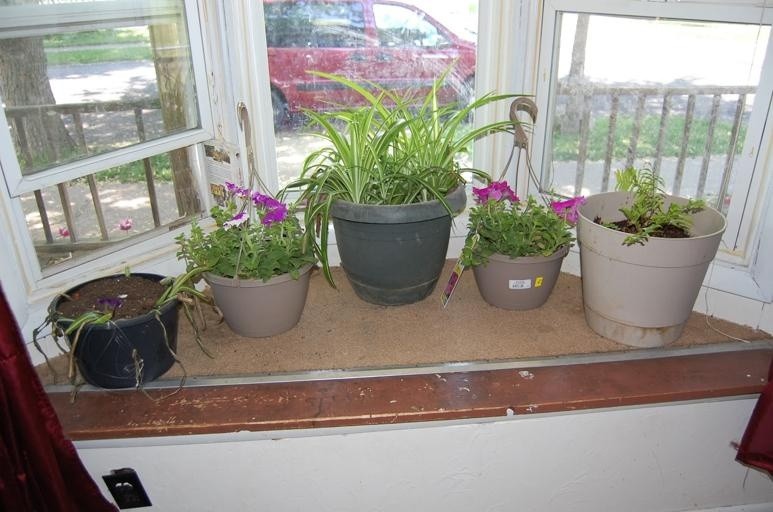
[575, 156, 726, 349]
[31, 272, 233, 389]
[276, 55, 518, 306]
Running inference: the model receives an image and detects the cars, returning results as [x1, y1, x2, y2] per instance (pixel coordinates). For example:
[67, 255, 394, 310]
[265, 0, 477, 132]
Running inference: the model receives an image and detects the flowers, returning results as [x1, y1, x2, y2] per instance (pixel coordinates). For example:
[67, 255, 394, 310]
[468, 180, 584, 259]
[176, 181, 322, 281]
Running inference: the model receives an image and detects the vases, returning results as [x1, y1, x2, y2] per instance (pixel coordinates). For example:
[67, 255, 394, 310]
[201, 262, 316, 339]
[467, 247, 569, 312]
